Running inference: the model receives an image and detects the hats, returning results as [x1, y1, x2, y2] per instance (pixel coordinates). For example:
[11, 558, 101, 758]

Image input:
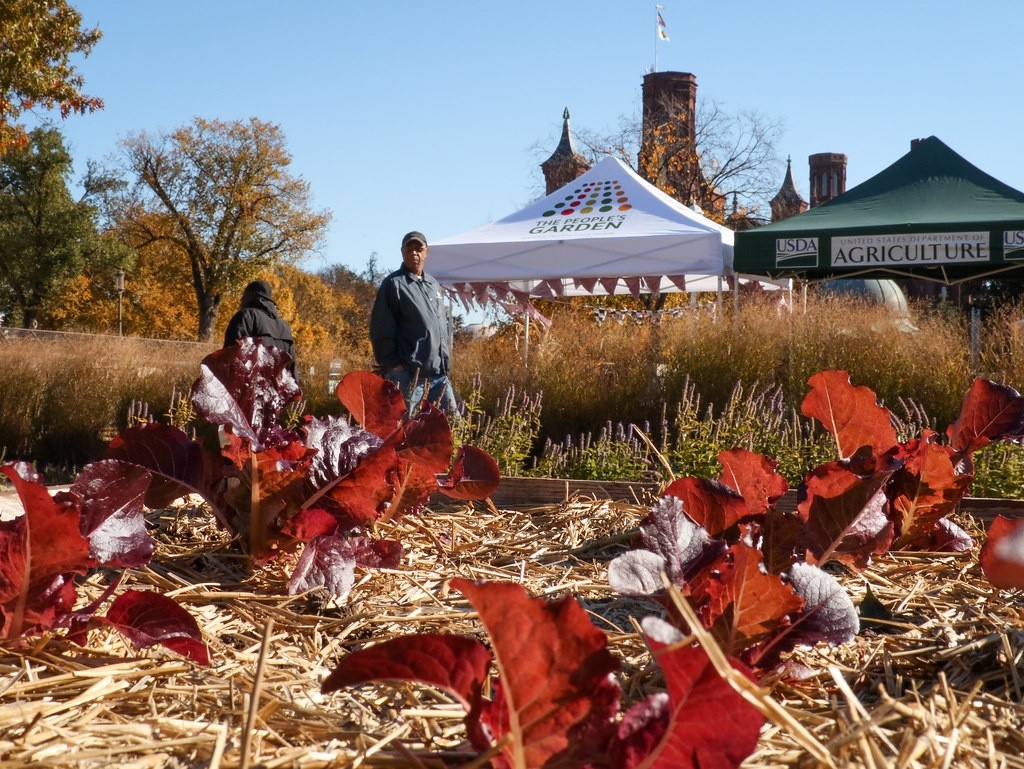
[245, 280, 278, 306]
[402, 232, 428, 249]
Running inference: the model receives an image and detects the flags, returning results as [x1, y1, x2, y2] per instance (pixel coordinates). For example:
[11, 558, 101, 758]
[658, 11, 671, 41]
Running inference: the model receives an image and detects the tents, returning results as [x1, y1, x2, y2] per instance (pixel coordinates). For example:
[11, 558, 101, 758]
[733, 135, 1024, 373]
[423, 135, 794, 373]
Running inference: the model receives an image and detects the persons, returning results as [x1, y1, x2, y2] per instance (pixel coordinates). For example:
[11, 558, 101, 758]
[369, 231, 464, 428]
[223, 280, 298, 386]
